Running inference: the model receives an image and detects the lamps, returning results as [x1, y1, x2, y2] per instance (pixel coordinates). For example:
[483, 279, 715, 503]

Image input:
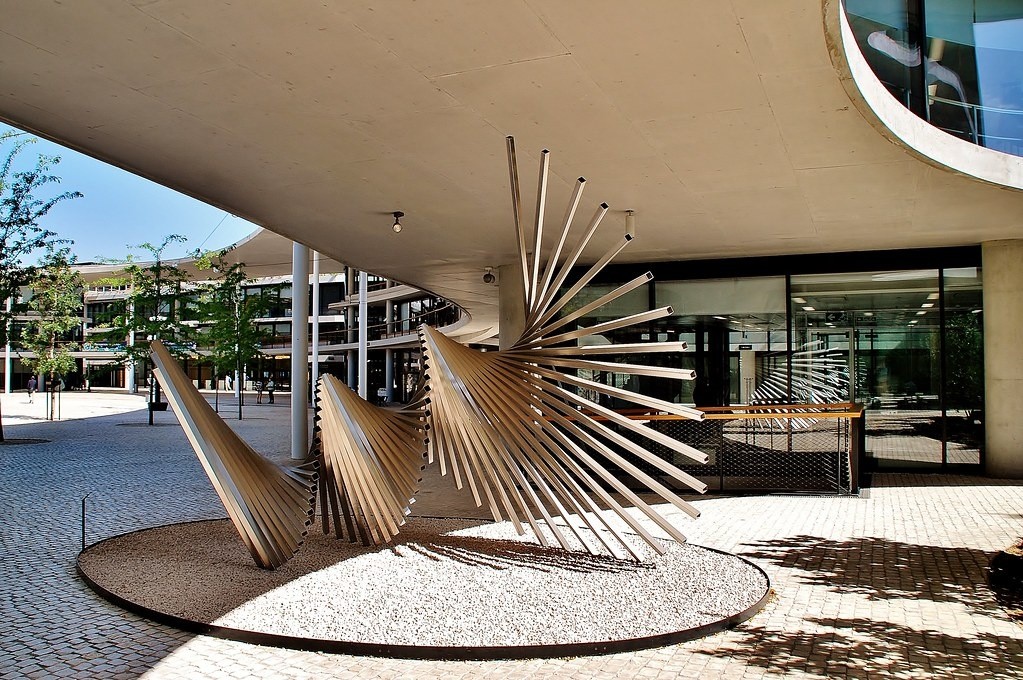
[483, 267, 495, 284]
[392, 210, 404, 233]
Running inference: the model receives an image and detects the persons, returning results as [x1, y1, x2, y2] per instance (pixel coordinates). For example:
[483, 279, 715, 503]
[225, 375, 232, 390]
[255, 378, 264, 404]
[27, 376, 37, 403]
[265, 378, 274, 404]
